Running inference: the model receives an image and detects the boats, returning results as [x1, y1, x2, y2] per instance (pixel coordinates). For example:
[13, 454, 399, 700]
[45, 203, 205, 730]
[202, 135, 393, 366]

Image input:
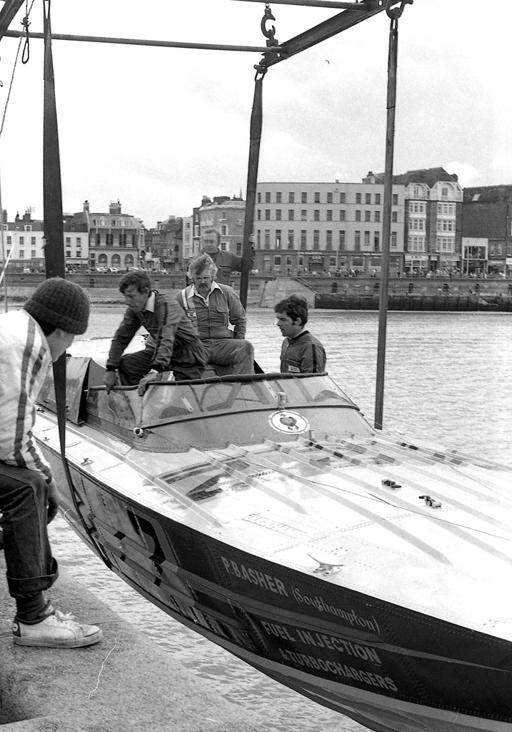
[32, 353, 511, 732]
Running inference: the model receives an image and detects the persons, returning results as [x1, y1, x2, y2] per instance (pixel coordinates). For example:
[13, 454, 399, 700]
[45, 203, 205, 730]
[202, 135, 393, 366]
[173, 250, 256, 379]
[273, 295, 326, 374]
[105, 271, 207, 398]
[1, 275, 104, 649]
[183, 227, 256, 284]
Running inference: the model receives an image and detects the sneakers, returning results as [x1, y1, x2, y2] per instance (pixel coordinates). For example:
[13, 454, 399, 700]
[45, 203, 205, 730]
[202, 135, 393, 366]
[11, 599, 105, 649]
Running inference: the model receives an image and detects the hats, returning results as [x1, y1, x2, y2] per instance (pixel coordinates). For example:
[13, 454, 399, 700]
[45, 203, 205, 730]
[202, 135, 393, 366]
[24, 274, 91, 335]
